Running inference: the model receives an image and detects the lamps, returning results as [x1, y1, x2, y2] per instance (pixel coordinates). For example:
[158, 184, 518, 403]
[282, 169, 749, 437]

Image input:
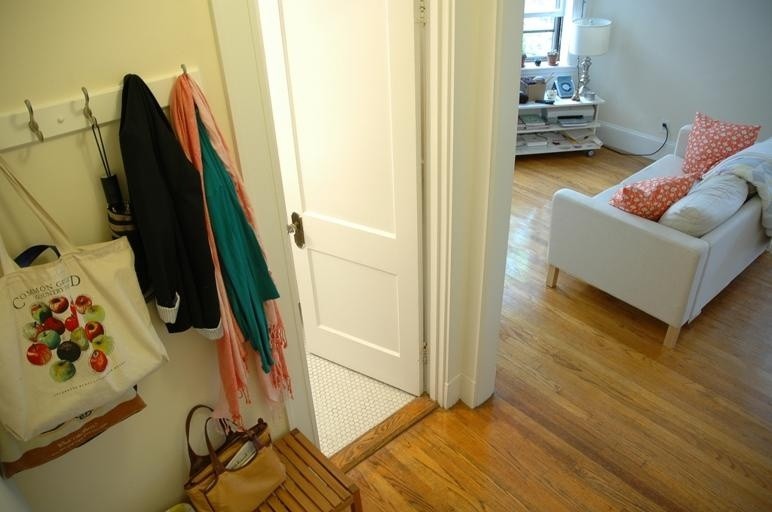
[570, 17, 612, 95]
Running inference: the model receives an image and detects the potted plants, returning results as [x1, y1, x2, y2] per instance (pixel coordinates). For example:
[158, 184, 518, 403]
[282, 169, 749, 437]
[546, 49, 558, 65]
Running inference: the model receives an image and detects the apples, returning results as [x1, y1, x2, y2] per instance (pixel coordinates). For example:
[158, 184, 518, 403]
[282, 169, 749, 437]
[65, 315, 79, 331]
[69, 326, 90, 350]
[92, 334, 115, 355]
[48, 296, 68, 313]
[84, 304, 107, 323]
[30, 302, 52, 321]
[49, 360, 75, 384]
[37, 329, 61, 350]
[86, 322, 104, 343]
[91, 349, 107, 371]
[70, 300, 76, 313]
[75, 295, 92, 314]
[26, 343, 52, 366]
[56, 341, 81, 362]
[43, 317, 65, 335]
[23, 321, 44, 342]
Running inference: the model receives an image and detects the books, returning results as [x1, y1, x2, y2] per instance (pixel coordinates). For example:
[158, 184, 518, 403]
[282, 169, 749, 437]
[515, 114, 597, 147]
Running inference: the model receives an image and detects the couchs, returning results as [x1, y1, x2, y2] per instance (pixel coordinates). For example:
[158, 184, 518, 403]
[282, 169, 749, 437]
[545, 124, 772, 352]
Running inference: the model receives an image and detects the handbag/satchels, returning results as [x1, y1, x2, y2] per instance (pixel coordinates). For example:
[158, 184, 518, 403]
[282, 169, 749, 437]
[185, 405, 286, 511]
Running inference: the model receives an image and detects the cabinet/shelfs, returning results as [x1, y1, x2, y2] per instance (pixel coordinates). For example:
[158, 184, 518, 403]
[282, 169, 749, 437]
[514, 92, 607, 158]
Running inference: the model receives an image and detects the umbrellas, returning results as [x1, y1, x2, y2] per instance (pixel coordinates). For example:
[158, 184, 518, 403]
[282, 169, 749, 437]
[98, 174, 150, 300]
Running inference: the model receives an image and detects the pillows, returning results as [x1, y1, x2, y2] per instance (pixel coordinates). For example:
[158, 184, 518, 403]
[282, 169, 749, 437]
[611, 113, 759, 219]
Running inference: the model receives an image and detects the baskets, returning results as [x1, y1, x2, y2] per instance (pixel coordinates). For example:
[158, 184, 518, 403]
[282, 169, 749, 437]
[520, 76, 546, 102]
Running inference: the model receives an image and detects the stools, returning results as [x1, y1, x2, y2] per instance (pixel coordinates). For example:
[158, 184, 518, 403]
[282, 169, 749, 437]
[239, 427, 364, 512]
